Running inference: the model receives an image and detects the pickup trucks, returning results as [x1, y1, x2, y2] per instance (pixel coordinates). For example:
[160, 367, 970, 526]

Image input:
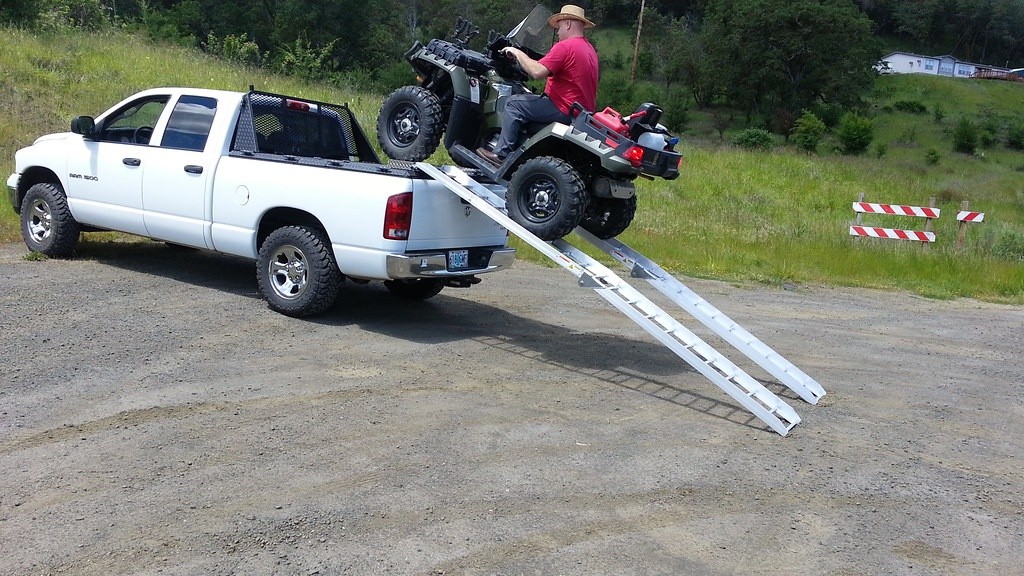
[3, 84, 517, 320]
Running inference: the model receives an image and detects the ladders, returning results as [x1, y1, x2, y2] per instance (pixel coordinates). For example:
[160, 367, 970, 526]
[453, 145, 827, 405]
[415, 162, 801, 435]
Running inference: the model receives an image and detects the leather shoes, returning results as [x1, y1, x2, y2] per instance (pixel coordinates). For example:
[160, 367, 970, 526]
[476, 147, 503, 168]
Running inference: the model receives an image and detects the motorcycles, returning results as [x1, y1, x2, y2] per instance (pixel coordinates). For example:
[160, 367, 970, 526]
[373, 2, 684, 244]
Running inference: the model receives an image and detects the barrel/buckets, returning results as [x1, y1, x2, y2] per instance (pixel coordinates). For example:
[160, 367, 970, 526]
[591, 107, 630, 138]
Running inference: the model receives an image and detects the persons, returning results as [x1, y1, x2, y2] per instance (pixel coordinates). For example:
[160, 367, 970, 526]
[475, 5, 598, 167]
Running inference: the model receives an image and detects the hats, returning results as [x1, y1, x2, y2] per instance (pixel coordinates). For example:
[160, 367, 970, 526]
[548, 4, 596, 28]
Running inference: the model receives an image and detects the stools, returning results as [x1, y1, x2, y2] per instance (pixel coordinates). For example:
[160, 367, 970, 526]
[525, 121, 551, 136]
